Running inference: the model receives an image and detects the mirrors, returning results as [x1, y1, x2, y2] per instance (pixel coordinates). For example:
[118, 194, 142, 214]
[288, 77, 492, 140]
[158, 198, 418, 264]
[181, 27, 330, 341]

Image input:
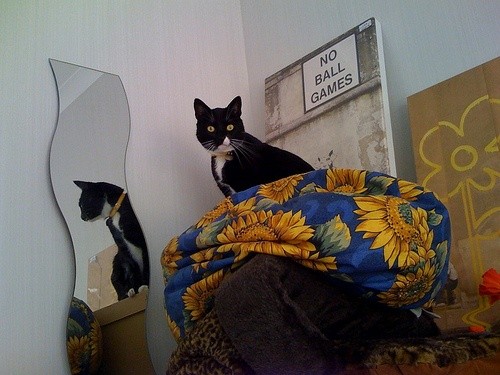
[50, 58, 156, 375]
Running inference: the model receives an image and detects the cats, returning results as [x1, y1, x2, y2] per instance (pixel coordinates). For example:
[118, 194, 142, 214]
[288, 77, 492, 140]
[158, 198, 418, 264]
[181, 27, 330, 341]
[74, 180, 150, 300]
[193, 96, 317, 197]
